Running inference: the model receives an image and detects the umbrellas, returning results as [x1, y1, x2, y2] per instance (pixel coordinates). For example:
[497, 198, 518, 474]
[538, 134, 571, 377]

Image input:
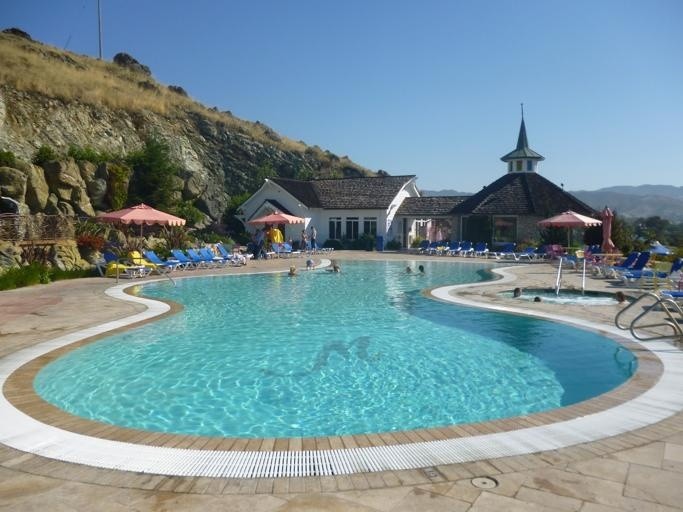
[246, 210, 305, 259]
[94, 203, 186, 265]
[602, 206, 615, 253]
[538, 210, 602, 248]
[641, 240, 675, 263]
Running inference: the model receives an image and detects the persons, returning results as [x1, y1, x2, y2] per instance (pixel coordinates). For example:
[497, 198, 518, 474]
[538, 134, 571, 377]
[418, 265, 426, 273]
[513, 287, 523, 297]
[616, 291, 630, 306]
[334, 265, 341, 272]
[534, 297, 542, 301]
[253, 227, 271, 259]
[300, 228, 307, 252]
[311, 226, 317, 253]
[288, 265, 298, 278]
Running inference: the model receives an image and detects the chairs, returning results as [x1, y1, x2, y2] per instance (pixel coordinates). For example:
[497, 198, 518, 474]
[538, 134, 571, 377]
[272, 241, 335, 258]
[90, 246, 252, 278]
[410, 240, 683, 298]
